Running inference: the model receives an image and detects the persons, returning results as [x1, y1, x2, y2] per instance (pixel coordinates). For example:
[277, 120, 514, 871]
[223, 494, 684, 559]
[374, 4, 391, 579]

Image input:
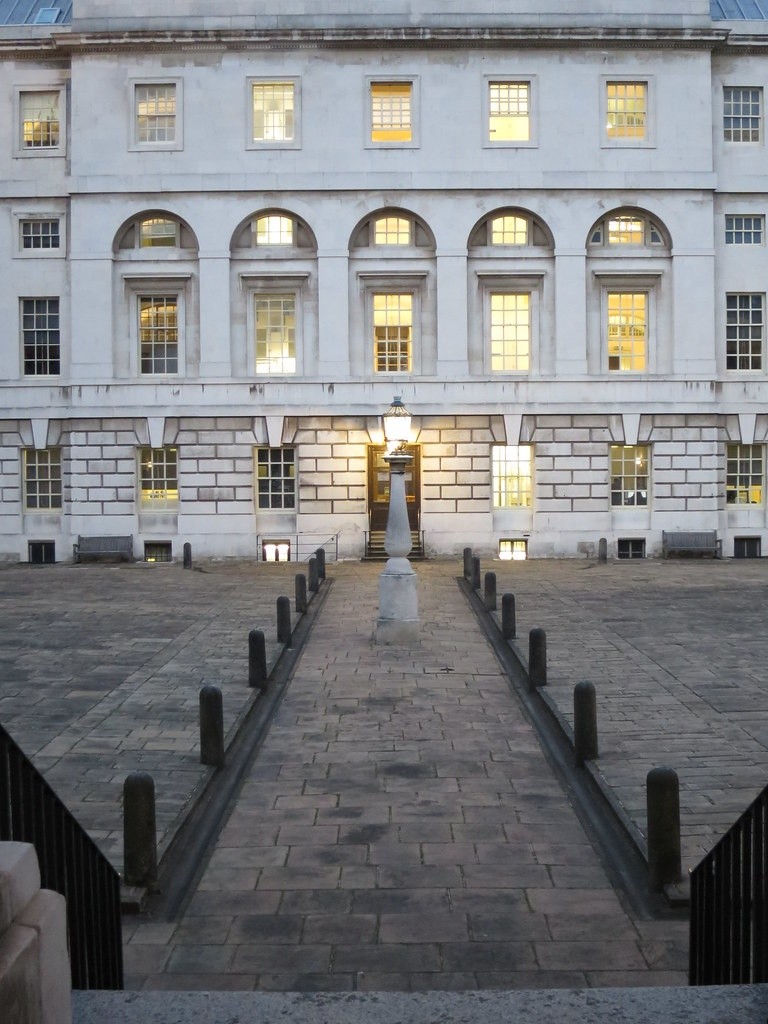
[612, 477, 621, 505]
